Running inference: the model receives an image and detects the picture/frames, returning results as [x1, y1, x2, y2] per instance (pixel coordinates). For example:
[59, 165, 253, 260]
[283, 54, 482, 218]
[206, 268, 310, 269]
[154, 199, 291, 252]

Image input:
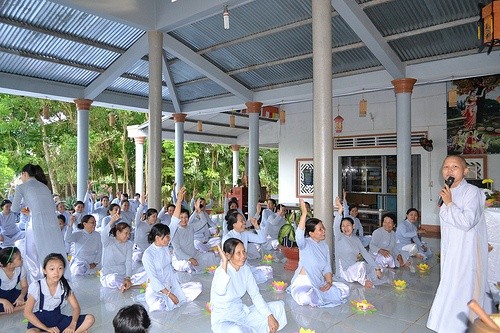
[296, 158, 313, 199]
[462, 154, 487, 180]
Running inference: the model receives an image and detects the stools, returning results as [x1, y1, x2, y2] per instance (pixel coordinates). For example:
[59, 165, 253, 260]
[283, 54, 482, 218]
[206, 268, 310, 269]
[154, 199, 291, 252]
[359, 223, 376, 235]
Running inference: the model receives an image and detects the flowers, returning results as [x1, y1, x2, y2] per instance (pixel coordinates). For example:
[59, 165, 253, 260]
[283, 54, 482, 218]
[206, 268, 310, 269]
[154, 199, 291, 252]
[482, 178, 500, 208]
[21, 244, 432, 333]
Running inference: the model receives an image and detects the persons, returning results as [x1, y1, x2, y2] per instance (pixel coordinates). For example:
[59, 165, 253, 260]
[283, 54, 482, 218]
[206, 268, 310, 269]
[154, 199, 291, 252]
[10, 163, 72, 295]
[426, 154, 490, 333]
[0, 181, 432, 333]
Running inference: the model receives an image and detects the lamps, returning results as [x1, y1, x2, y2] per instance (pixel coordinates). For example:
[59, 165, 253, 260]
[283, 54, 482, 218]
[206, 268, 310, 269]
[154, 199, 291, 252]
[359, 88, 367, 117]
[279, 100, 285, 125]
[229, 108, 235, 128]
[477, 0, 500, 55]
[198, 120, 202, 132]
[334, 98, 344, 133]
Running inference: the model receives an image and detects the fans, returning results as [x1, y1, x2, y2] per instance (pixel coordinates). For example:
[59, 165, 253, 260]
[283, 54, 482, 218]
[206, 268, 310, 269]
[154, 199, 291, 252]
[418, 137, 434, 153]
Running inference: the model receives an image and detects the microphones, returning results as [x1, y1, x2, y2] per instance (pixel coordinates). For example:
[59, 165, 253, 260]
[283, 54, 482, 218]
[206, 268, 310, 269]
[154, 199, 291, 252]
[437, 176, 455, 207]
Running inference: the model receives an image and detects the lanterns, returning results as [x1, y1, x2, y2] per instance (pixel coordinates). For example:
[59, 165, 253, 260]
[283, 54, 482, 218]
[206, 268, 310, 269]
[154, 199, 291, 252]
[477, 0, 500, 55]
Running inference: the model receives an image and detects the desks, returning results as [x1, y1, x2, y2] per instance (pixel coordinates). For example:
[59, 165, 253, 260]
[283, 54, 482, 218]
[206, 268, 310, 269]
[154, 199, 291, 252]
[256, 203, 314, 215]
[483, 207, 500, 303]
[357, 208, 396, 227]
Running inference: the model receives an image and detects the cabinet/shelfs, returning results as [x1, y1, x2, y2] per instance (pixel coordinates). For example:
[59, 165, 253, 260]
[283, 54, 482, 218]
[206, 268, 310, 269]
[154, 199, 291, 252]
[225, 186, 267, 212]
[346, 166, 382, 192]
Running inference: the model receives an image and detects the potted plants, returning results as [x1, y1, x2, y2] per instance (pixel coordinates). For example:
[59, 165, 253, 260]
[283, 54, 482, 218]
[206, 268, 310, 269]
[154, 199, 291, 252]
[277, 212, 313, 271]
